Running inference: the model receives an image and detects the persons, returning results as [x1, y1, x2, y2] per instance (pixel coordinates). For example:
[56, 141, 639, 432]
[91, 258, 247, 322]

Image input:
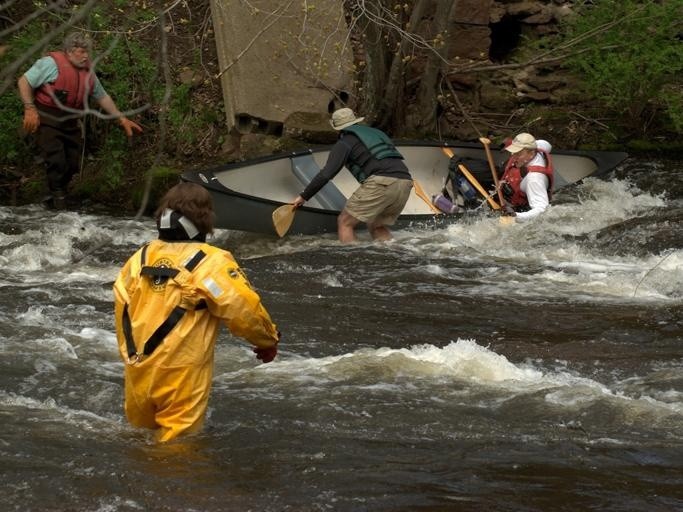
[17, 32, 142, 210]
[498, 133, 555, 223]
[294, 108, 413, 243]
[111, 182, 279, 457]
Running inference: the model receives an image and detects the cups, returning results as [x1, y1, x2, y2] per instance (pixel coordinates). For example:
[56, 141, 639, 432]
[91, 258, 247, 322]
[432, 194, 459, 214]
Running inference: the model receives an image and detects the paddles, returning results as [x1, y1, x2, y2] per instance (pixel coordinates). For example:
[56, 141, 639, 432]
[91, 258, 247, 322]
[272, 204, 296, 237]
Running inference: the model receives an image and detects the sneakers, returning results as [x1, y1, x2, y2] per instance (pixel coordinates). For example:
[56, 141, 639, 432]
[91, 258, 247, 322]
[52, 191, 77, 210]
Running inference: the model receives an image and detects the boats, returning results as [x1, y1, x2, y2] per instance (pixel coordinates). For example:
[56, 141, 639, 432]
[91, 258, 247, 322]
[179, 141, 628, 235]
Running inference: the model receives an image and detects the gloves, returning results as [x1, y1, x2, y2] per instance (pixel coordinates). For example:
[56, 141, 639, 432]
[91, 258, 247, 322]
[113, 117, 144, 137]
[500, 207, 517, 217]
[253, 346, 277, 363]
[23, 103, 41, 134]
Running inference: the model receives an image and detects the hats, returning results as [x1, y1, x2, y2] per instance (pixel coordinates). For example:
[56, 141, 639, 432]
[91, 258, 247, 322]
[504, 132, 537, 153]
[330, 108, 365, 130]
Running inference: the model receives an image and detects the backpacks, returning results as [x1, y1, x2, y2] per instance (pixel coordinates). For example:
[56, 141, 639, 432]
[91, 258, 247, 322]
[443, 156, 500, 209]
